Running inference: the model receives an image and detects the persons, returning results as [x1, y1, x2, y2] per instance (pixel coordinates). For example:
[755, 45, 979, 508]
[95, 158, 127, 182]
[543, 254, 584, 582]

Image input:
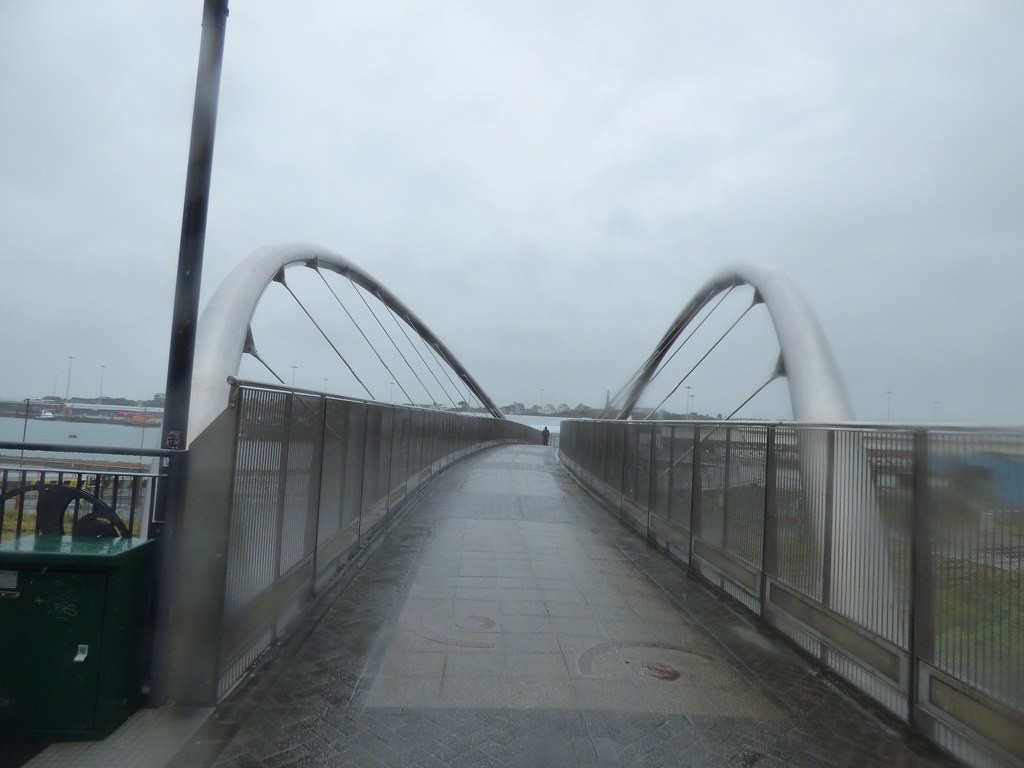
[542, 427, 550, 446]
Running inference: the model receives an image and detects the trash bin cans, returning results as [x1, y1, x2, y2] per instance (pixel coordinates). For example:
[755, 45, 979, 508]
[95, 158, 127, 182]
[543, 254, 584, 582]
[0, 532, 156, 742]
